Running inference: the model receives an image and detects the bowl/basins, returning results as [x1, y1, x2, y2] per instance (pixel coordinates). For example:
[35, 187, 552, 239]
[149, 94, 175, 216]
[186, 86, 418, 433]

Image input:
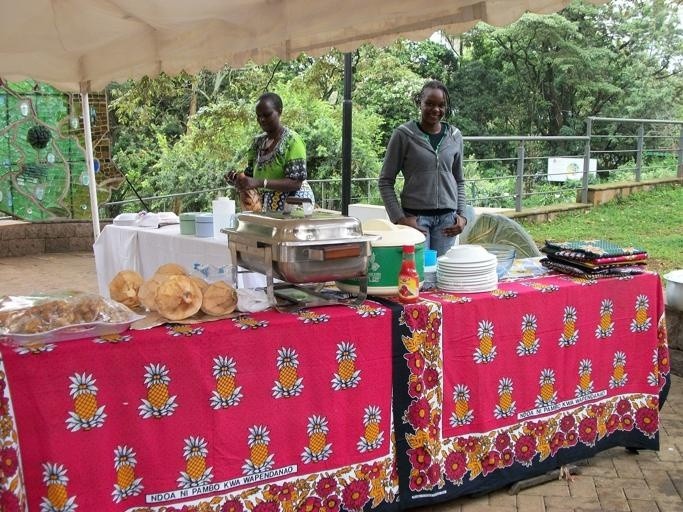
[484, 247, 517, 278]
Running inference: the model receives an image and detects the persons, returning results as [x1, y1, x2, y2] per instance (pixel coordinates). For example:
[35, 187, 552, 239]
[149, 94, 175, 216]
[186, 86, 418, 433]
[377, 80, 469, 260]
[224, 93, 316, 215]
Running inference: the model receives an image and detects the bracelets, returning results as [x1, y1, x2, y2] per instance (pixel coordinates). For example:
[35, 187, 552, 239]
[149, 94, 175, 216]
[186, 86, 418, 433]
[263, 178, 267, 189]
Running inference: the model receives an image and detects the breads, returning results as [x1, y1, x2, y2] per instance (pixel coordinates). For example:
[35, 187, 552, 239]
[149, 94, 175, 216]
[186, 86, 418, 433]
[109, 263, 238, 321]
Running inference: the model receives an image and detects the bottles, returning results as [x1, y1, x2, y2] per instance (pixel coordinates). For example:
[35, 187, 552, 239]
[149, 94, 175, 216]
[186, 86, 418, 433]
[398, 244, 420, 306]
[211, 197, 236, 241]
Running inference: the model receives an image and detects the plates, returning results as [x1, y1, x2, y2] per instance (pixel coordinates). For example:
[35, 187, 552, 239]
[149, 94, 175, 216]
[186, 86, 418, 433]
[435, 244, 499, 294]
